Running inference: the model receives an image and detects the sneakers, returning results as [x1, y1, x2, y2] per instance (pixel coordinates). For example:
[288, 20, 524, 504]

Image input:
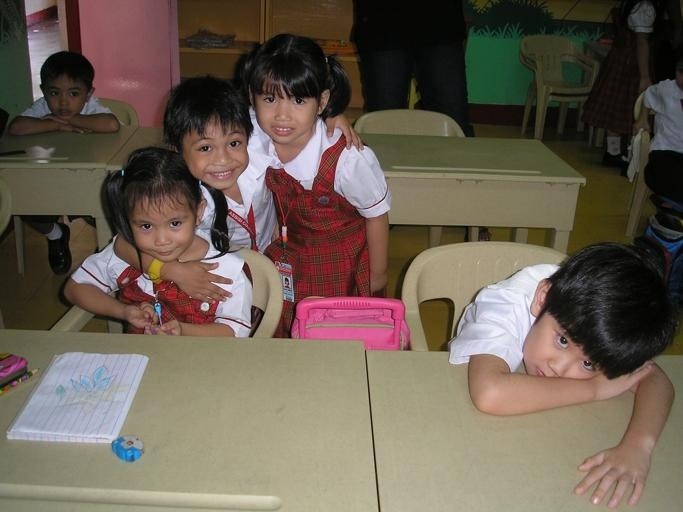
[45, 222, 71, 276]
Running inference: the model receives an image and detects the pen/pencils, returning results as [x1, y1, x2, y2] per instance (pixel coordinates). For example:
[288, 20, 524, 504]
[0, 150, 26, 158]
[0, 366, 40, 397]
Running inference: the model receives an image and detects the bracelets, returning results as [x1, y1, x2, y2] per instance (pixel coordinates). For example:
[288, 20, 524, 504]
[145, 257, 165, 286]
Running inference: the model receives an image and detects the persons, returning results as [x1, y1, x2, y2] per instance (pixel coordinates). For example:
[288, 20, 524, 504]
[112, 74, 369, 339]
[348, 1, 493, 241]
[61, 145, 255, 339]
[228, 32, 392, 338]
[631, 41, 683, 207]
[283, 277, 290, 288]
[446, 240, 677, 509]
[5, 51, 127, 277]
[579, 0, 670, 176]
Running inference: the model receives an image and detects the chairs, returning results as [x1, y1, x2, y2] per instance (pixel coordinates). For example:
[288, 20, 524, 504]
[518, 34, 600, 144]
[351, 108, 481, 248]
[12, 97, 138, 277]
